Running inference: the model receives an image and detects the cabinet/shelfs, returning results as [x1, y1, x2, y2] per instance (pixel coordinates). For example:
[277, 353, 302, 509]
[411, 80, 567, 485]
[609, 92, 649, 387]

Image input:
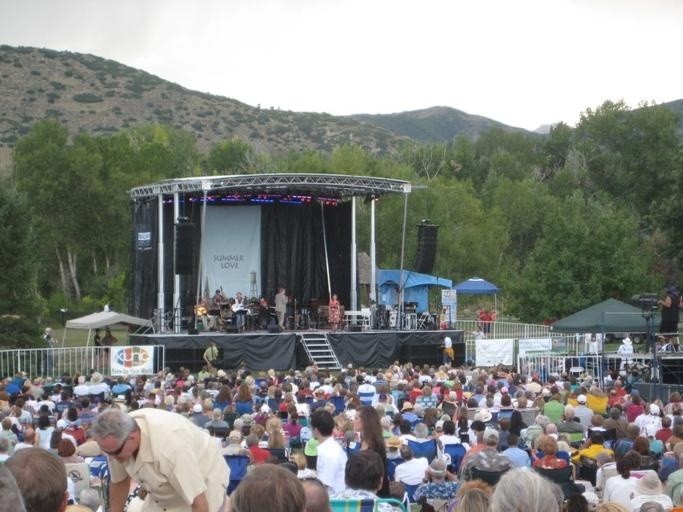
[342, 311, 371, 331]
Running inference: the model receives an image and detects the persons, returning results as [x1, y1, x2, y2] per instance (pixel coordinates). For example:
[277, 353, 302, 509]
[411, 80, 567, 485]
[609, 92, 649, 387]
[436, 333, 454, 367]
[102, 328, 118, 370]
[616, 337, 633, 354]
[39, 327, 59, 377]
[193, 287, 316, 332]
[0, 359, 682, 511]
[656, 283, 681, 353]
[327, 293, 341, 331]
[466, 308, 499, 339]
[202, 341, 220, 366]
[93, 328, 104, 369]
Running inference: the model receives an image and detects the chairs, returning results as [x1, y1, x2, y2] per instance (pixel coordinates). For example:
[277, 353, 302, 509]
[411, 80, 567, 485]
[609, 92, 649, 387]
[417, 312, 428, 330]
[0, 377, 683, 512]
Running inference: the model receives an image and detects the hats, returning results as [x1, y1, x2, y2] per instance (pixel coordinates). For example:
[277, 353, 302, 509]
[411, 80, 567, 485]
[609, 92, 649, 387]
[482, 428, 500, 445]
[379, 395, 414, 448]
[57, 419, 70, 430]
[435, 421, 444, 431]
[474, 410, 493, 423]
[576, 394, 587, 403]
[634, 472, 662, 495]
[541, 388, 551, 398]
[165, 395, 269, 442]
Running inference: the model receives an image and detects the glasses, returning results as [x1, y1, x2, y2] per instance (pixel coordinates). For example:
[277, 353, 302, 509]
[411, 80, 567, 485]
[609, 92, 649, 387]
[105, 438, 129, 456]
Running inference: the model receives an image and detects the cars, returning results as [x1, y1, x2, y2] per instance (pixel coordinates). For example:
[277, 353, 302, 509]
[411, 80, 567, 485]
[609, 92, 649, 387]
[605, 331, 646, 344]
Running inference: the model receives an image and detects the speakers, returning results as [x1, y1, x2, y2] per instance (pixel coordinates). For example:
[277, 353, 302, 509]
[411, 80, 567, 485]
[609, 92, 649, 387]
[175, 223, 198, 274]
[414, 225, 440, 273]
[406, 314, 418, 331]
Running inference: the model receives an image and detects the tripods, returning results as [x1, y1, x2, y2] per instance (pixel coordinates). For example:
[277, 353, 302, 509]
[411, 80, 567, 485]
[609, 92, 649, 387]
[620, 312, 682, 391]
